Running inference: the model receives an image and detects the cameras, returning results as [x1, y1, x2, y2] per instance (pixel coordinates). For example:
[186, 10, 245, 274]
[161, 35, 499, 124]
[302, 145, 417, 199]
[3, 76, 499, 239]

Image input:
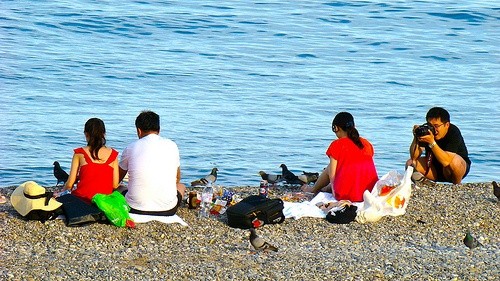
[416, 122, 436, 138]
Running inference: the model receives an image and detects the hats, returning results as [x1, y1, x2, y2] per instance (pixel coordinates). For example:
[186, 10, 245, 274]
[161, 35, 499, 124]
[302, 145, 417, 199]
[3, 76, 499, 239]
[11, 180, 63, 216]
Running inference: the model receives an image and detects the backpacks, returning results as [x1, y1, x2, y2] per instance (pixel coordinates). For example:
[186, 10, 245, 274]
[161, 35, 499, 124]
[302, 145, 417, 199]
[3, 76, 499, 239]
[228, 195, 286, 229]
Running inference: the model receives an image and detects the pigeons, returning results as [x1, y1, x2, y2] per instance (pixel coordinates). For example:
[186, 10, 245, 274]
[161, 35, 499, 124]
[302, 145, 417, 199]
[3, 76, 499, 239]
[279, 164, 306, 185]
[491, 181, 500, 201]
[258, 171, 286, 187]
[52, 161, 77, 187]
[190, 168, 220, 187]
[464, 230, 484, 250]
[247, 228, 278, 255]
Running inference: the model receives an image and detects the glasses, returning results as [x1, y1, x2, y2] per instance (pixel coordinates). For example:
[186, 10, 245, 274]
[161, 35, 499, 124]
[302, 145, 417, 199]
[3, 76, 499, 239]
[434, 123, 443, 128]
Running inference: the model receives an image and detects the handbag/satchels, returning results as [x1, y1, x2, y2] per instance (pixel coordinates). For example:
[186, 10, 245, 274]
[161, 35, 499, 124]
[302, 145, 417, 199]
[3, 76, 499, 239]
[364, 166, 413, 222]
[91, 191, 131, 228]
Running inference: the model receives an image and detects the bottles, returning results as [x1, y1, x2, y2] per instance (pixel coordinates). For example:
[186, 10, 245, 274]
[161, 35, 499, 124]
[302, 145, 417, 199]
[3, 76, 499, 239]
[200, 181, 213, 218]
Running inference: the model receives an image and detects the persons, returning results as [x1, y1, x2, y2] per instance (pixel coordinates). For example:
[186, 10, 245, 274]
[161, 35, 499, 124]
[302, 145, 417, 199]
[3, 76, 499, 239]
[118, 111, 186, 217]
[300, 112, 379, 202]
[62, 118, 122, 204]
[405, 107, 471, 185]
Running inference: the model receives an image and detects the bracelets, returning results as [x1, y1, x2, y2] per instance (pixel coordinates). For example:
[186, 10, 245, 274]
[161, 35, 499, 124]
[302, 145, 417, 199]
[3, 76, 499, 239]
[429, 141, 437, 148]
